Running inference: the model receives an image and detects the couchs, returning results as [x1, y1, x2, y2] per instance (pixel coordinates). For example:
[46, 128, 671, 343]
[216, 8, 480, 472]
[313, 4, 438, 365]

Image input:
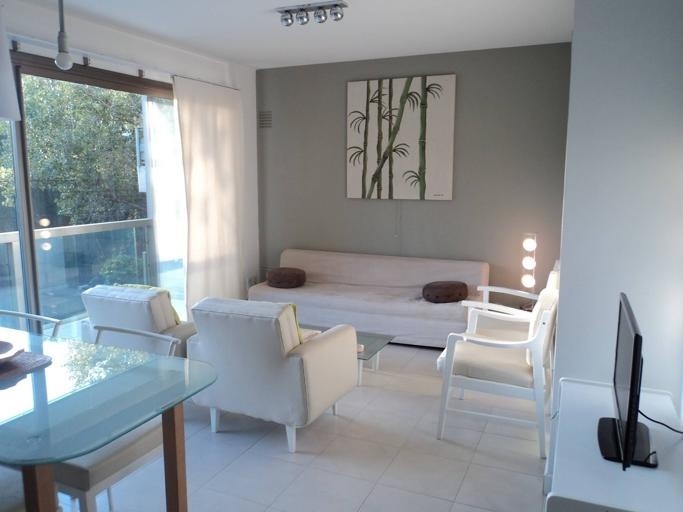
[246, 248, 490, 351]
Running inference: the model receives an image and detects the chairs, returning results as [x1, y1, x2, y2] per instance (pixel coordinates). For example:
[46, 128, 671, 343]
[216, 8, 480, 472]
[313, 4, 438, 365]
[185, 293, 360, 456]
[80, 280, 197, 357]
[458, 259, 561, 411]
[435, 268, 560, 461]
[0, 309, 63, 340]
[53, 323, 181, 512]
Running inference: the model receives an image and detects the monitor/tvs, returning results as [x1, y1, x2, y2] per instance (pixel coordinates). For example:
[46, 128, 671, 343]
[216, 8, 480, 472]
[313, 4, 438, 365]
[597, 291, 659, 471]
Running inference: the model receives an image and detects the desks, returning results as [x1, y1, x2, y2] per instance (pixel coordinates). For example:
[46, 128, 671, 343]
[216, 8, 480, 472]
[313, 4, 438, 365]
[0, 325, 220, 512]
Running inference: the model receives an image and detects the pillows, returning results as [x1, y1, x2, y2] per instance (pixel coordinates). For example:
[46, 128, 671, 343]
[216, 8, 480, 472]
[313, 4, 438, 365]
[266, 267, 307, 289]
[421, 279, 469, 304]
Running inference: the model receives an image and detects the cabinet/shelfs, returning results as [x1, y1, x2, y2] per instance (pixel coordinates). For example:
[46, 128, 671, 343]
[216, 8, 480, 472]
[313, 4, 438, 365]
[544, 374, 683, 511]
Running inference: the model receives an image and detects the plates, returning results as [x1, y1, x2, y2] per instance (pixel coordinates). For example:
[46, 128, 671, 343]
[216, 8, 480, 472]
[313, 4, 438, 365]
[0, 338, 25, 364]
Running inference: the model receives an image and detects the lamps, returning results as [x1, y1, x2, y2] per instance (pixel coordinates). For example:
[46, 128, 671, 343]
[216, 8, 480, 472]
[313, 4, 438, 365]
[55, 1, 74, 74]
[274, 0, 350, 27]
[521, 228, 538, 310]
